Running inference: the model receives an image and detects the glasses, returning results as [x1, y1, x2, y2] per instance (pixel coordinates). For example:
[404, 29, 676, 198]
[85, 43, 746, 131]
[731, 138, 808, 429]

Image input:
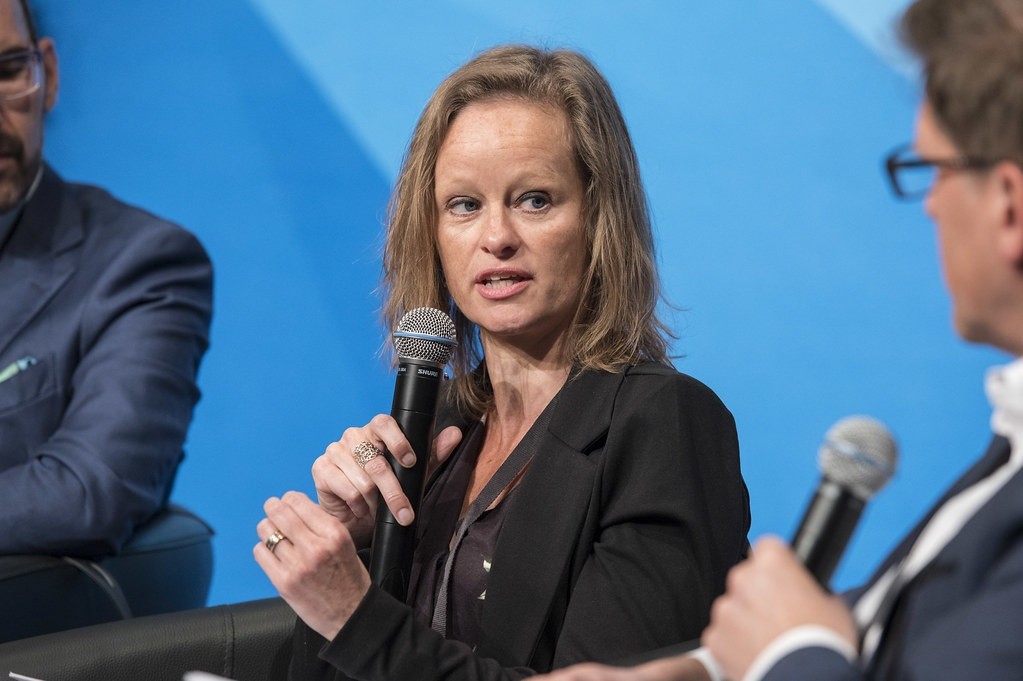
[884, 143, 971, 201]
[0, 50, 43, 101]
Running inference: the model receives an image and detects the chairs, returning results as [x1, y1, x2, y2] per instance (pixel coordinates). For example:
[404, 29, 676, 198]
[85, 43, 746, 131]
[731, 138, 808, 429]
[0, 501, 299, 681]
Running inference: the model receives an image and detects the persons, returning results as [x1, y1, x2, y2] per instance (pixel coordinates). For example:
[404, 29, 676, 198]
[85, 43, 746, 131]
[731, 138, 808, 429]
[252, 46, 754, 681]
[0, 2, 213, 558]
[526, 0, 1023, 681]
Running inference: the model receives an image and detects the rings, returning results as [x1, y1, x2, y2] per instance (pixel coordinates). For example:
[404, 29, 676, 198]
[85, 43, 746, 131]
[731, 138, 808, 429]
[352, 441, 384, 470]
[266, 530, 286, 552]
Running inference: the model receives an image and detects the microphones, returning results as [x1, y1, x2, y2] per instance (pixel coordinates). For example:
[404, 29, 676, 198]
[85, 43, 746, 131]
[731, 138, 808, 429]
[786, 413, 899, 586]
[367, 308, 458, 605]
